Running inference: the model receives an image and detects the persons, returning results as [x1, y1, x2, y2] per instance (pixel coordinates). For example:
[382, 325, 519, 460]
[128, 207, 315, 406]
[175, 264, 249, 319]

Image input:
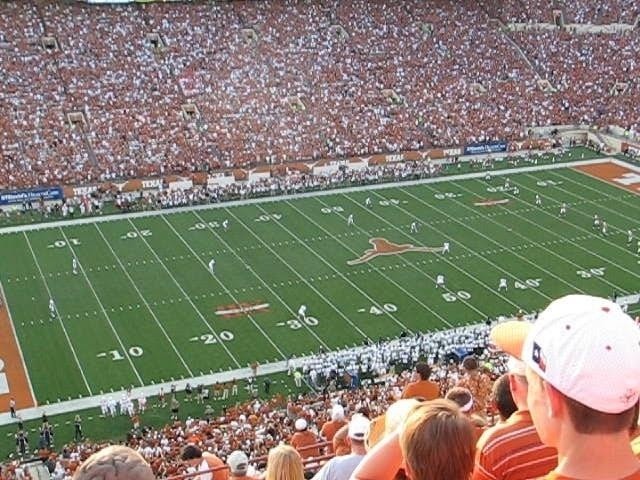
[298, 304, 307, 319]
[411, 220, 418, 233]
[442, 242, 450, 255]
[498, 278, 508, 292]
[209, 258, 216, 273]
[223, 220, 228, 228]
[347, 213, 354, 226]
[49, 298, 55, 311]
[72, 258, 77, 270]
[628, 230, 633, 243]
[601, 220, 608, 232]
[593, 214, 600, 230]
[490, 295, 639, 477]
[436, 275, 445, 289]
[1, 294, 637, 478]
[0, 0, 640, 216]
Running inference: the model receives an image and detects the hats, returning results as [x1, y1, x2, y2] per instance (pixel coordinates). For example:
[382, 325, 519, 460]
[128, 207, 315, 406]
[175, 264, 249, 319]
[295, 419, 307, 430]
[348, 414, 371, 440]
[331, 405, 344, 421]
[444, 387, 472, 412]
[492, 295, 639, 414]
[227, 450, 249, 473]
